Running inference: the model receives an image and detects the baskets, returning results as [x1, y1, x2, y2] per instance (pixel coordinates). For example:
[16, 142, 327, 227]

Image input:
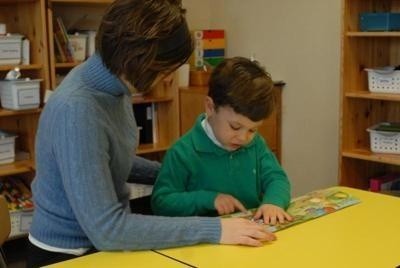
[366, 121, 400, 154]
[363, 65, 400, 94]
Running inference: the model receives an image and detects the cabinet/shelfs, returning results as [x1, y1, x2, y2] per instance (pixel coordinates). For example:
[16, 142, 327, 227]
[0, 0, 182, 241]
[179, 81, 287, 171]
[339, 0, 400, 197]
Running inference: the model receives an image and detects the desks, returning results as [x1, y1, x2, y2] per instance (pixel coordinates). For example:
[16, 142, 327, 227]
[34, 185, 400, 268]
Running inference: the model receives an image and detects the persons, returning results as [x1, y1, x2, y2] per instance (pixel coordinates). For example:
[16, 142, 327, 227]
[148, 55, 294, 227]
[22, 1, 276, 267]
